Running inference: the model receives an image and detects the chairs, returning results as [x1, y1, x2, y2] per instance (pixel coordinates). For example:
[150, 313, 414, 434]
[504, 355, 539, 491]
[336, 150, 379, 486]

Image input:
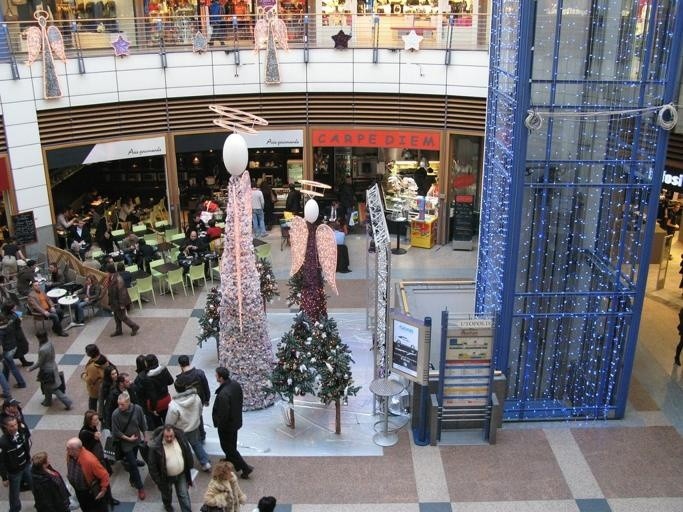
[279, 219, 290, 251]
[0, 196, 272, 331]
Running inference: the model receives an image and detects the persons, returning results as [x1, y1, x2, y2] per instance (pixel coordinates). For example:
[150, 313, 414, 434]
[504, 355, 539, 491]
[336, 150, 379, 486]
[0, 187, 220, 512]
[341, 177, 354, 210]
[257, 497, 277, 511]
[213, 367, 253, 478]
[251, 184, 270, 238]
[674, 309, 683, 366]
[286, 183, 301, 214]
[261, 181, 276, 229]
[332, 225, 349, 246]
[206, 462, 247, 512]
[663, 200, 681, 243]
[323, 202, 342, 223]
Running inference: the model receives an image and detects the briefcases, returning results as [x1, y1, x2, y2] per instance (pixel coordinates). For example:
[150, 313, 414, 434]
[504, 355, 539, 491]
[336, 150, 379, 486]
[41, 371, 65, 394]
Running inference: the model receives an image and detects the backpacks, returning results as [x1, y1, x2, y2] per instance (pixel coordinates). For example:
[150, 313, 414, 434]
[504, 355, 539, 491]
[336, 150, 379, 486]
[183, 380, 205, 403]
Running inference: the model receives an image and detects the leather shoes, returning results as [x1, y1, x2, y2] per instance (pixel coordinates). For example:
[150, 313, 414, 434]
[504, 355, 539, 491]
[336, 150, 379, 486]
[111, 499, 119, 505]
[138, 487, 145, 500]
[131, 326, 139, 336]
[111, 332, 123, 337]
[241, 469, 251, 479]
[22, 362, 33, 367]
[136, 460, 145, 467]
[57, 333, 68, 337]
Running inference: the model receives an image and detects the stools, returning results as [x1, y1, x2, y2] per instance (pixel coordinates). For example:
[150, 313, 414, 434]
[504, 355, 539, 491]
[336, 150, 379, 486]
[386, 213, 408, 255]
[370, 379, 406, 448]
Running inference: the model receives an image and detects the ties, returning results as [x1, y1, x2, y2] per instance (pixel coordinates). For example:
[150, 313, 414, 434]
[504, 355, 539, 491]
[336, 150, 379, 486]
[333, 207, 335, 218]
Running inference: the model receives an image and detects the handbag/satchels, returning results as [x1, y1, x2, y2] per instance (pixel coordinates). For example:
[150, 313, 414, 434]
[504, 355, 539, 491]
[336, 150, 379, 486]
[199, 501, 223, 512]
[105, 437, 120, 456]
[39, 369, 55, 384]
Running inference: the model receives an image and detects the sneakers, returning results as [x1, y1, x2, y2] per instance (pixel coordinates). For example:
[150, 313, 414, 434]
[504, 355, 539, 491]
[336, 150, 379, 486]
[0, 382, 25, 397]
[201, 463, 210, 472]
